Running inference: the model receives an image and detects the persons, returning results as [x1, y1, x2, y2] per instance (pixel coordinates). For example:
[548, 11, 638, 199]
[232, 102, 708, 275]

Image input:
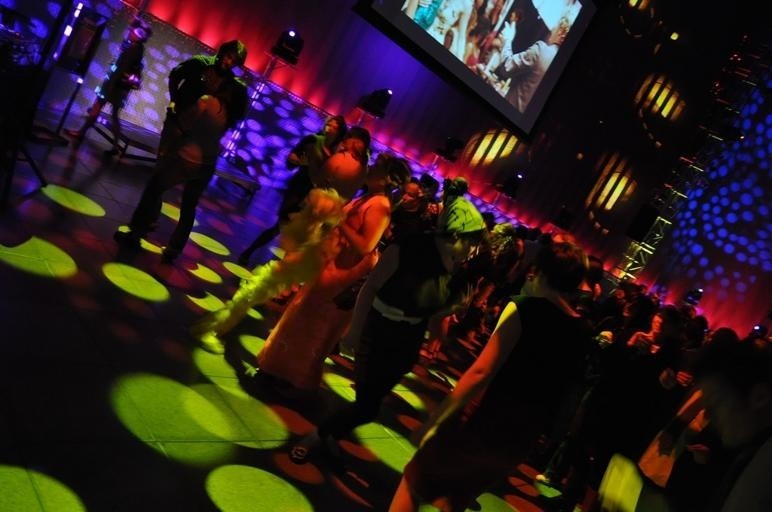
[400, 0, 572, 114]
[115, 40, 248, 260]
[67, 28, 147, 154]
[186, 115, 772, 511]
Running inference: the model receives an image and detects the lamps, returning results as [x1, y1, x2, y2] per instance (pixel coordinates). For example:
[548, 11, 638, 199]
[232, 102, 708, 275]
[749, 325, 768, 339]
[436, 136, 467, 161]
[270, 28, 305, 65]
[683, 287, 705, 305]
[358, 87, 394, 117]
[503, 172, 523, 199]
